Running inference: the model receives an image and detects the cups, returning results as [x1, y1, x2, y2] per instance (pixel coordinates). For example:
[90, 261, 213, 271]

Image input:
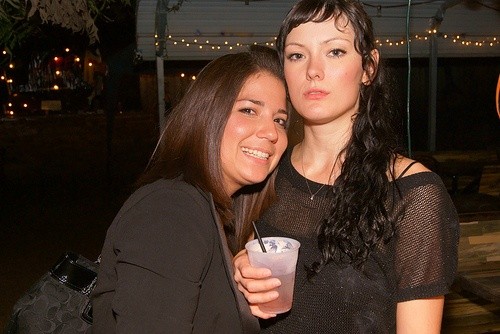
[243, 237, 301, 316]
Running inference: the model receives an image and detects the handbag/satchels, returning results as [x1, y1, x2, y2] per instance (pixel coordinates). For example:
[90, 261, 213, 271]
[7, 251, 101, 334]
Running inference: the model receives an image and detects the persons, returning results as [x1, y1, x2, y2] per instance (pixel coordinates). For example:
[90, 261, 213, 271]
[91, 42, 292, 334]
[228, 1, 461, 334]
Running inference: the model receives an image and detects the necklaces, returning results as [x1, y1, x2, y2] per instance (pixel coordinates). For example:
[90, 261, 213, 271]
[298, 141, 340, 200]
